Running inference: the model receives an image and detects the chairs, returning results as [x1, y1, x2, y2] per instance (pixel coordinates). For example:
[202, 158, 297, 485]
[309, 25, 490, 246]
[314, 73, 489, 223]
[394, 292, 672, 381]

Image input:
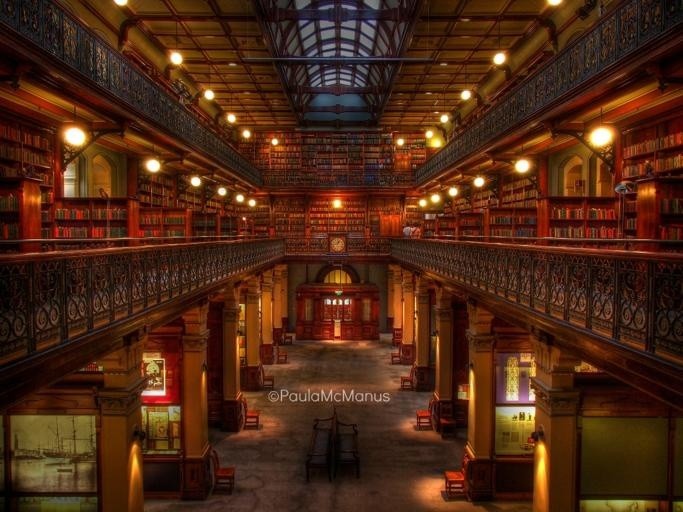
[400, 366, 415, 391]
[390, 343, 402, 365]
[260, 363, 274, 391]
[415, 398, 435, 430]
[443, 447, 470, 500]
[439, 400, 456, 438]
[209, 448, 234, 495]
[282, 330, 292, 345]
[276, 338, 288, 364]
[241, 395, 263, 431]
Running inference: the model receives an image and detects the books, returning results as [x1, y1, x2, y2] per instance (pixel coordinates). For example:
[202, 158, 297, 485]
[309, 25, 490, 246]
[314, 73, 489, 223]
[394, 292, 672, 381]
[0, 127, 682, 313]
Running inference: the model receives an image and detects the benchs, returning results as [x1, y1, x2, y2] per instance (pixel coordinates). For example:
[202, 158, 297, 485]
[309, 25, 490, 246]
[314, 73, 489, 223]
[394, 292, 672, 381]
[335, 406, 363, 482]
[304, 406, 335, 484]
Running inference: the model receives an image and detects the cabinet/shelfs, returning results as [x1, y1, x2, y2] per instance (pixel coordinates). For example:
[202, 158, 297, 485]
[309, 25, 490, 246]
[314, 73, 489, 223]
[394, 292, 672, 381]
[406, 43, 681, 249]
[0, 48, 272, 262]
[274, 130, 404, 236]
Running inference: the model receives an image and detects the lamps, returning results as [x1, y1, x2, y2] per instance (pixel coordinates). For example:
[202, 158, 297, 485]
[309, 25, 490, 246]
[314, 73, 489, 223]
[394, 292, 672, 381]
[130, 428, 148, 443]
[528, 423, 544, 449]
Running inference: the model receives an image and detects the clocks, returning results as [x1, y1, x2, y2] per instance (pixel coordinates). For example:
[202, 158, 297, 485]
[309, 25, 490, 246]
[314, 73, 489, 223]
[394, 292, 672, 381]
[328, 236, 347, 256]
